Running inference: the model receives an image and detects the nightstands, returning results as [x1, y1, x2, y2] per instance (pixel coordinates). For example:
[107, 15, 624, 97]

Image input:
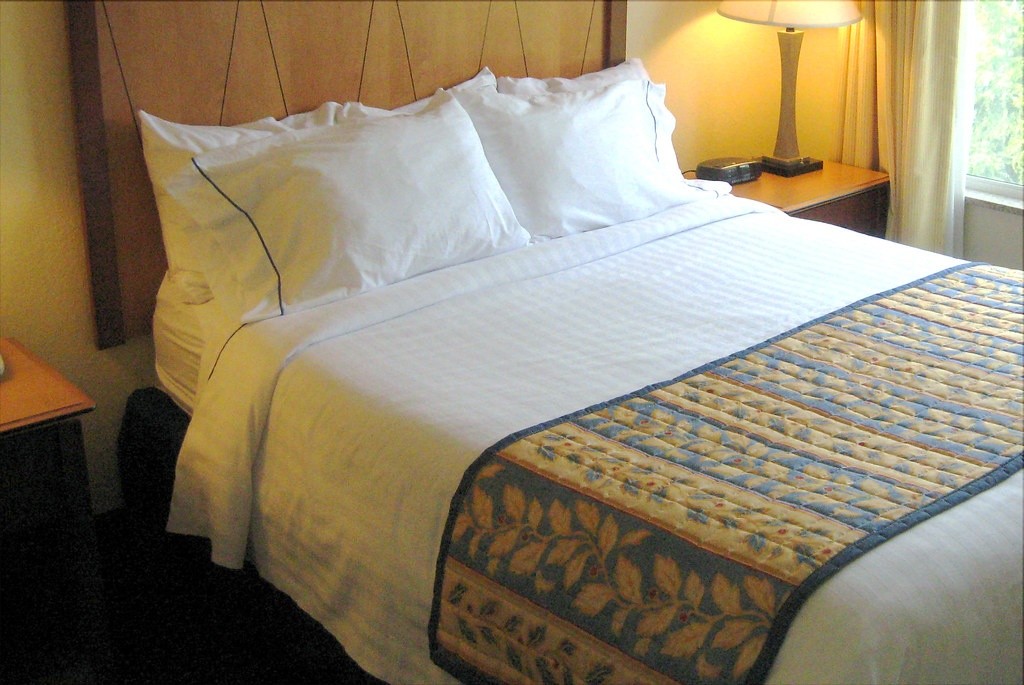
[687, 163, 891, 237]
[0, 335, 113, 685]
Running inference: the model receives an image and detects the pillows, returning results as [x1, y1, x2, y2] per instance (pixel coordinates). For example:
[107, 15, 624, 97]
[139, 57, 737, 324]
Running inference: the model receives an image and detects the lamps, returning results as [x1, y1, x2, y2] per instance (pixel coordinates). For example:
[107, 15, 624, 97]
[718, 0, 862, 178]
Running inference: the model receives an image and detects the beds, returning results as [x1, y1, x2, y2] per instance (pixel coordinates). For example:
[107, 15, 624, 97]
[126, 199, 1024, 685]
